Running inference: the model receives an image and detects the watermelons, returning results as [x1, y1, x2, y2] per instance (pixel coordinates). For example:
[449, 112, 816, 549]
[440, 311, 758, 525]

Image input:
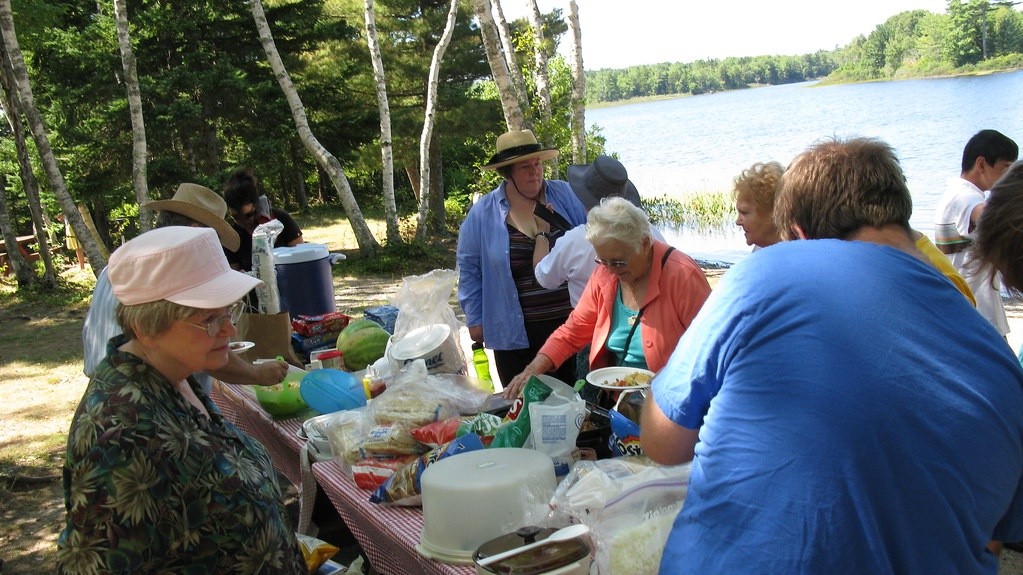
[337, 318, 389, 371]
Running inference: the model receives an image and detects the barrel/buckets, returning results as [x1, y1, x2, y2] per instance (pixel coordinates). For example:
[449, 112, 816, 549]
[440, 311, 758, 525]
[392, 324, 466, 377]
[272, 243, 346, 322]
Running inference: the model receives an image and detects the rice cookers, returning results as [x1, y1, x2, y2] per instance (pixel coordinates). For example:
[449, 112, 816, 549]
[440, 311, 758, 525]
[472, 527, 591, 575]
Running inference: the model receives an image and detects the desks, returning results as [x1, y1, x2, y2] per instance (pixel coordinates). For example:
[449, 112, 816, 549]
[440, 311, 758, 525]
[212, 353, 478, 574]
[0, 235, 36, 277]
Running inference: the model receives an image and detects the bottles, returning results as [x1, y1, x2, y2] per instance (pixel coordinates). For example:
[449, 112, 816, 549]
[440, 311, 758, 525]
[471, 342, 496, 393]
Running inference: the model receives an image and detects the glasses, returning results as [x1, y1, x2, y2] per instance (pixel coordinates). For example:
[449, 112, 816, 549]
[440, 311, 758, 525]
[595, 247, 635, 268]
[227, 204, 256, 221]
[176, 300, 246, 339]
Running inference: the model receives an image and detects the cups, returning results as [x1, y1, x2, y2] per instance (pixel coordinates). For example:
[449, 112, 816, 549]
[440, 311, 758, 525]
[252, 233, 279, 315]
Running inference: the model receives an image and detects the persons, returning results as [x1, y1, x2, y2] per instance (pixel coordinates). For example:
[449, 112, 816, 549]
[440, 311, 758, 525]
[80, 171, 304, 404]
[458, 129, 586, 389]
[906, 225, 979, 307]
[733, 161, 785, 252]
[933, 129, 1017, 343]
[640, 133, 1023, 575]
[61, 225, 311, 575]
[961, 157, 1023, 371]
[504, 198, 711, 415]
[534, 155, 666, 406]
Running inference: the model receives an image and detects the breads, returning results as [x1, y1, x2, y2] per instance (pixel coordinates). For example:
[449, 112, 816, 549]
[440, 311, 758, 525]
[362, 396, 438, 457]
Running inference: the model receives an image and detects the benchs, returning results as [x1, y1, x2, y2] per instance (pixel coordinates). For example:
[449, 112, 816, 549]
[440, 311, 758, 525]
[0, 251, 39, 276]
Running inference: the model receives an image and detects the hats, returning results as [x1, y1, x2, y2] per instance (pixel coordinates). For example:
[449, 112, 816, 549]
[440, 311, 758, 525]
[139, 181, 240, 252]
[479, 127, 559, 170]
[566, 154, 640, 211]
[107, 226, 264, 310]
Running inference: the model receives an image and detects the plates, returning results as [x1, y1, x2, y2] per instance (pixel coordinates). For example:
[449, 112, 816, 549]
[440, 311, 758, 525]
[586, 367, 655, 391]
[227, 341, 255, 353]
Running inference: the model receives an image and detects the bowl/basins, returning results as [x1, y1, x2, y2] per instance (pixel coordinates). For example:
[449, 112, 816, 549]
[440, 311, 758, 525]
[252, 370, 309, 416]
[415, 447, 558, 565]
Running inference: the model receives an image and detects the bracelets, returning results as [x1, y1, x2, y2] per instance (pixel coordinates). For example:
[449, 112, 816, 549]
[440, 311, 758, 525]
[534, 232, 550, 239]
